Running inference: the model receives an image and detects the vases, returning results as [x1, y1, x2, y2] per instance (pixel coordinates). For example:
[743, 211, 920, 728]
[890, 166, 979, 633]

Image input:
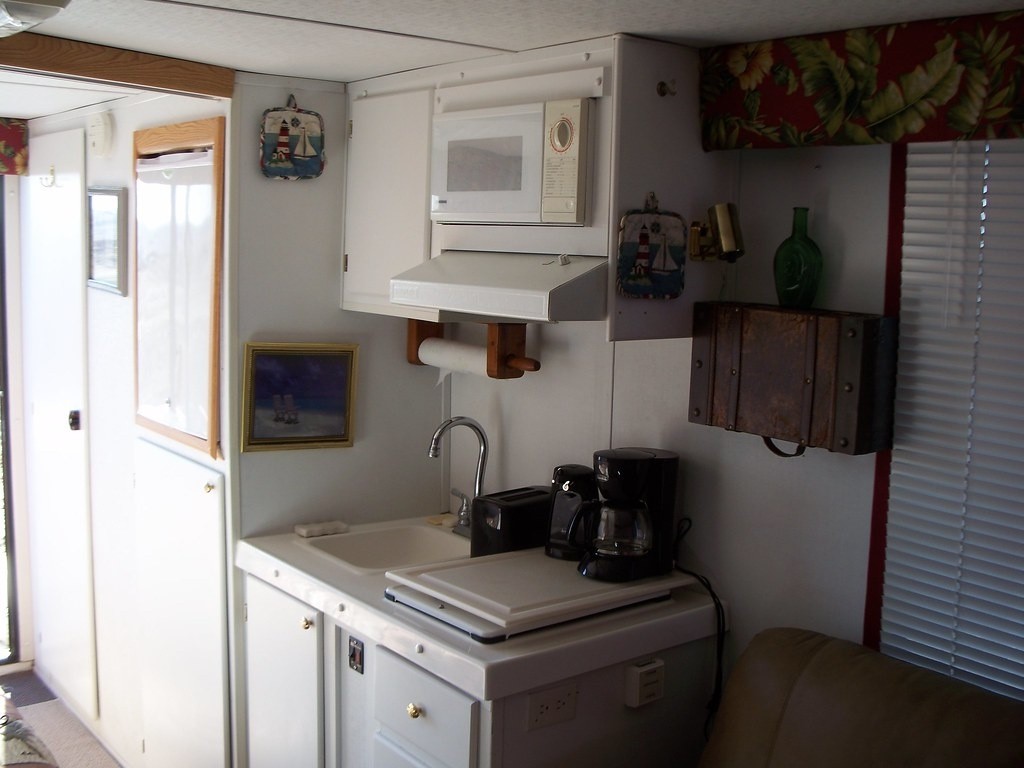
[771, 206, 824, 310]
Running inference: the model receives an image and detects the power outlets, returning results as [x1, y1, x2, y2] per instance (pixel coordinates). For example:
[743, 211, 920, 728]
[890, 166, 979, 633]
[525, 682, 576, 731]
[622, 656, 665, 708]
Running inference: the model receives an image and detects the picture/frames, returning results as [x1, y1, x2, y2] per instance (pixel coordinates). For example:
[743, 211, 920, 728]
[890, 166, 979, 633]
[258, 93, 326, 181]
[133, 115, 225, 462]
[84, 185, 129, 298]
[615, 191, 688, 300]
[239, 343, 359, 454]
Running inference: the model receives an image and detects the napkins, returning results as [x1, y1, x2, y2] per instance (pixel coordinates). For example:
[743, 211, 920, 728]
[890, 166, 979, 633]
[418, 336, 487, 389]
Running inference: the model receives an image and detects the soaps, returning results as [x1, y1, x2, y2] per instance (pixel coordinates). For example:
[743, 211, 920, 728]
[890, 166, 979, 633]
[292, 519, 353, 540]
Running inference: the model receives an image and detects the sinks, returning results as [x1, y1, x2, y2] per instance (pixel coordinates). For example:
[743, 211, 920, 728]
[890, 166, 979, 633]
[296, 520, 472, 579]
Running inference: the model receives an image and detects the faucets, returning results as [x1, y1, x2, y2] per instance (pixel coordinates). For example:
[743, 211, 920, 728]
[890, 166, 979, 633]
[428, 413, 492, 534]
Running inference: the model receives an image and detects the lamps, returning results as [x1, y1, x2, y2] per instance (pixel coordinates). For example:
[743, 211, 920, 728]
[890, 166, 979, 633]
[0, 0, 64, 38]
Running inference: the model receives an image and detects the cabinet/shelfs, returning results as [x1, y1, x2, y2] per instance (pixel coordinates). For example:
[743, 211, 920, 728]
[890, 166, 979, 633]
[242, 573, 731, 768]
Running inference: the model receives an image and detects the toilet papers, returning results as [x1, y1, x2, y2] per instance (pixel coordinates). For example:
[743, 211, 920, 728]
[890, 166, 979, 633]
[417, 334, 502, 392]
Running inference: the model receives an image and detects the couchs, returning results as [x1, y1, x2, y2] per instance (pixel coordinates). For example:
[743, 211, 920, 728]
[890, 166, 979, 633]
[703, 625, 1024, 767]
